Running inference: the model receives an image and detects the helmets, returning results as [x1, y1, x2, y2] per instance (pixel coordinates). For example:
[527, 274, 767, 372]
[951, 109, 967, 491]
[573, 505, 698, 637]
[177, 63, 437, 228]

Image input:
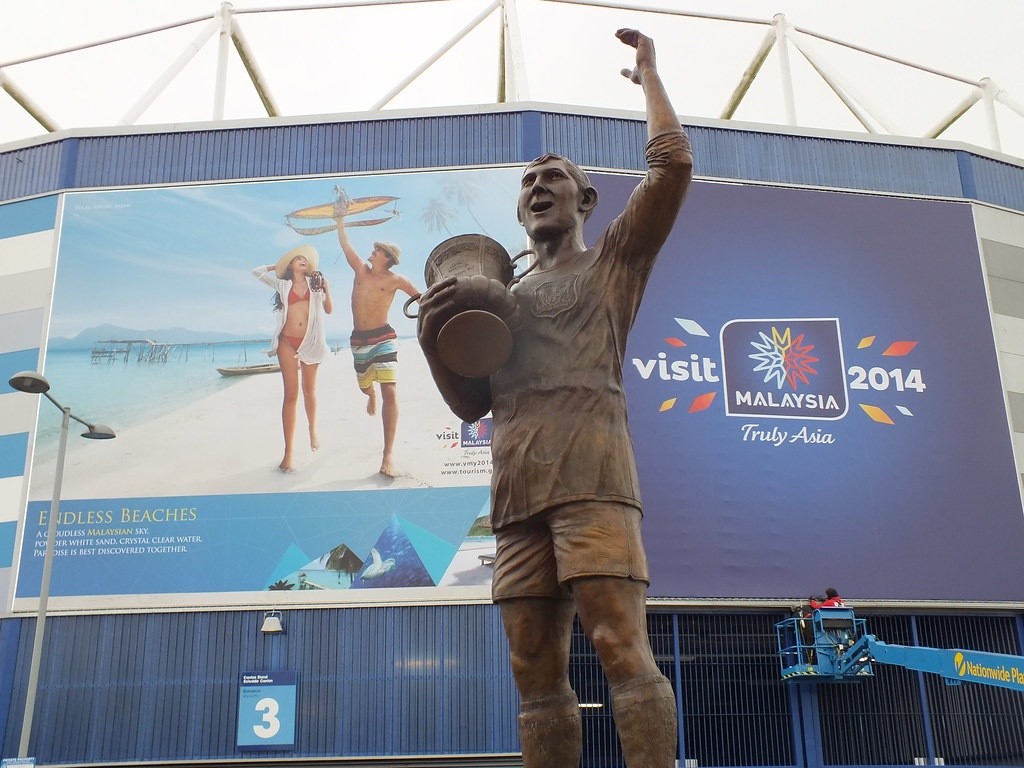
[826, 588, 839, 600]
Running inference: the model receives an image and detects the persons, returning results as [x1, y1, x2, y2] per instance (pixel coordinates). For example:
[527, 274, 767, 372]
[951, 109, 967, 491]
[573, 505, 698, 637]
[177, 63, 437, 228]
[416, 28, 693, 768]
[250, 245, 333, 475]
[333, 215, 423, 478]
[808, 588, 846, 608]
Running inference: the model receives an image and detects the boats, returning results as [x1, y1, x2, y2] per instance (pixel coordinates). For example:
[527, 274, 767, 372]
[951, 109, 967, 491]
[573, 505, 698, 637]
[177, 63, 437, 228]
[214, 364, 280, 375]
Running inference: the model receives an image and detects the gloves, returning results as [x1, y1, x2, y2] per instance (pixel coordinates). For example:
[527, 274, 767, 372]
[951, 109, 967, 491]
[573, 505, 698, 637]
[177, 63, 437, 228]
[810, 595, 815, 600]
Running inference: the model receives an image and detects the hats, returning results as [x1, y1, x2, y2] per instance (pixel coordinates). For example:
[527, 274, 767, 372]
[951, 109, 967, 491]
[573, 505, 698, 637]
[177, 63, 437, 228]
[374, 241, 401, 265]
[274, 245, 319, 279]
[801, 605, 812, 614]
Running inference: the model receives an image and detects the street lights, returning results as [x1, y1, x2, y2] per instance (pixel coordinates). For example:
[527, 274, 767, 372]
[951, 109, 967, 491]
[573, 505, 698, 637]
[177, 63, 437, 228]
[8, 371, 118, 758]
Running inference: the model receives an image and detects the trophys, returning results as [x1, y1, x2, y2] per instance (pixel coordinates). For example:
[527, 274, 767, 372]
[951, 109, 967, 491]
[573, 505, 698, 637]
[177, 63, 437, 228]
[403, 233, 541, 381]
